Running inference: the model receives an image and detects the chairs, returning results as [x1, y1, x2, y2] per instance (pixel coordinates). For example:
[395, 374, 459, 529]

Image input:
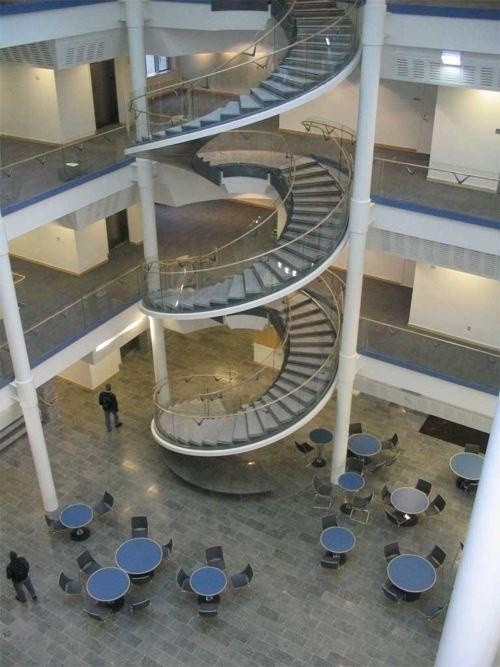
[295, 423, 485, 619]
[44, 491, 254, 622]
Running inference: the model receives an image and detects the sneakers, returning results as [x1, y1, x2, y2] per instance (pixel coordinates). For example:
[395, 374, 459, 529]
[107, 422, 121, 432]
[15, 596, 37, 602]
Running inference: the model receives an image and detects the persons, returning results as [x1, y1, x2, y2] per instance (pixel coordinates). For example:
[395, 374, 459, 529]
[5, 550, 38, 603]
[98, 383, 122, 431]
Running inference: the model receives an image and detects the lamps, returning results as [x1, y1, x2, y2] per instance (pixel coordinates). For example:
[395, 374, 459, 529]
[440, 50, 463, 65]
